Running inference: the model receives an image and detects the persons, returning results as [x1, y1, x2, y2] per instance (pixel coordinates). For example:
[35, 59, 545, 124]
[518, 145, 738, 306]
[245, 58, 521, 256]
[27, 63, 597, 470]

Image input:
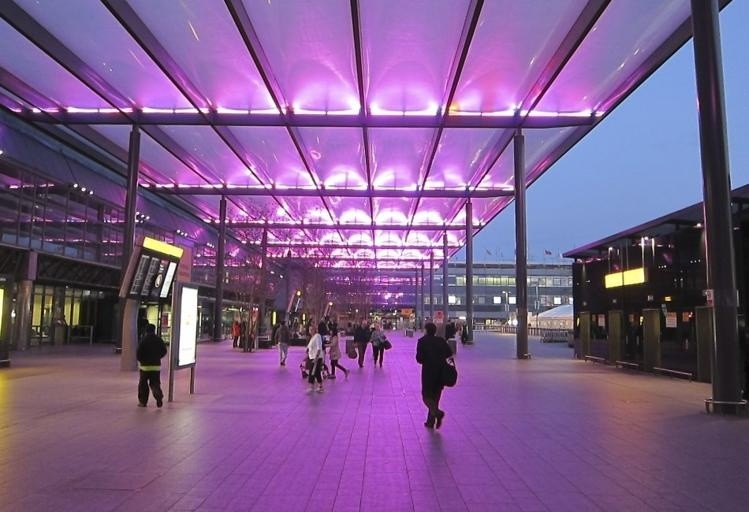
[445, 319, 469, 345]
[137, 315, 149, 345]
[135, 324, 167, 406]
[416, 323, 453, 428]
[304, 315, 386, 395]
[288, 318, 294, 329]
[231, 315, 256, 353]
[294, 319, 298, 329]
[274, 321, 290, 366]
[273, 320, 281, 331]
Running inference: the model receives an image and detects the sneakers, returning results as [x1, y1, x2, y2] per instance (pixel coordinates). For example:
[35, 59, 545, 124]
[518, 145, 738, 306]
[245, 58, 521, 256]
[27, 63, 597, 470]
[423, 422, 434, 427]
[436, 410, 444, 429]
[137, 402, 148, 407]
[157, 395, 162, 407]
[327, 374, 336, 379]
[306, 386, 325, 394]
[345, 368, 350, 377]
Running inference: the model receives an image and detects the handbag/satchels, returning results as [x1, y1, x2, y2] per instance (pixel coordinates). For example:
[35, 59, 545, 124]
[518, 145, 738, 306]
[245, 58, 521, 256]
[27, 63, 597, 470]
[300, 355, 310, 372]
[383, 340, 392, 350]
[348, 343, 357, 359]
[445, 357, 458, 388]
